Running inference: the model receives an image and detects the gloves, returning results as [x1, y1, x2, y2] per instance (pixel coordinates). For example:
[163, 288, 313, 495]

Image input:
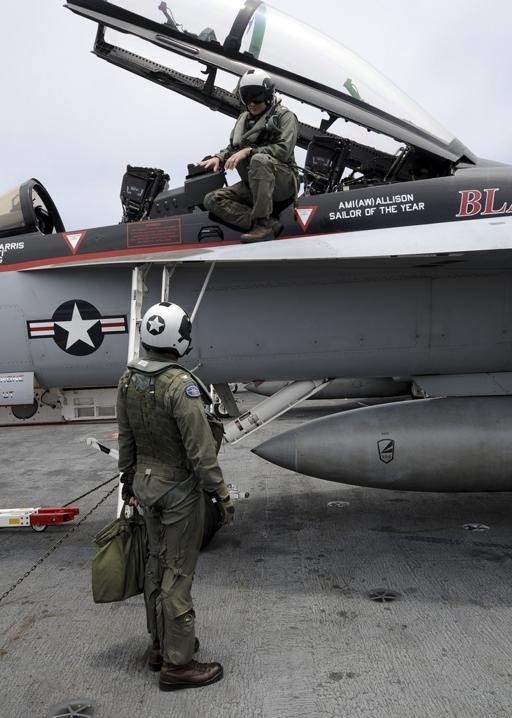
[210, 486, 235, 527]
[120, 472, 137, 507]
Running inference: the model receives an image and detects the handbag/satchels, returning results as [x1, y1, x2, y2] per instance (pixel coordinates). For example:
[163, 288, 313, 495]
[92, 503, 149, 603]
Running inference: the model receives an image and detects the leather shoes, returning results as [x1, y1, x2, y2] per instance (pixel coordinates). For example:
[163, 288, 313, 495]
[241, 216, 284, 242]
[159, 660, 223, 692]
[148, 637, 199, 672]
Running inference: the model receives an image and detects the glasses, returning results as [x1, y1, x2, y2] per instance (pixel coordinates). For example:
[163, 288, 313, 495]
[243, 92, 267, 106]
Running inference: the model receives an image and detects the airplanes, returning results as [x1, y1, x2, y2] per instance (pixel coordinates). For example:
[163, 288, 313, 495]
[0, 0, 512, 493]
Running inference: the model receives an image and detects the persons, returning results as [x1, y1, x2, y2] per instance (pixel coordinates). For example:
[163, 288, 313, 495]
[116, 300, 235, 695]
[196, 63, 299, 244]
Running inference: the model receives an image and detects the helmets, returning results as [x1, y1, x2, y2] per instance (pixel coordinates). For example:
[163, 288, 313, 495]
[239, 69, 275, 106]
[141, 301, 192, 358]
